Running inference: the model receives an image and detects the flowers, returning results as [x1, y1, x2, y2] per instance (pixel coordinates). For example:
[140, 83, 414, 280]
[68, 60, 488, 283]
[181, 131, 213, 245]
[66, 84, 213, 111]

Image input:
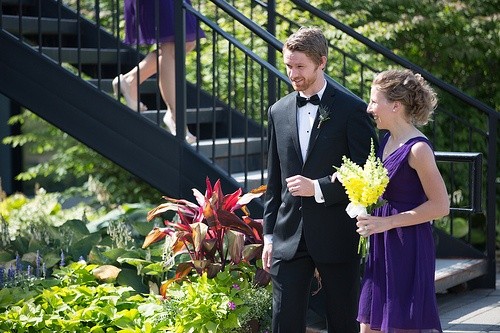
[332, 138, 389, 257]
[317, 104, 331, 128]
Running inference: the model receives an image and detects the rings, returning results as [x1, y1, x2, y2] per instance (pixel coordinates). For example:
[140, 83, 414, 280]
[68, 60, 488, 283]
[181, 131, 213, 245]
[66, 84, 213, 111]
[291, 188, 295, 192]
[364, 226, 367, 231]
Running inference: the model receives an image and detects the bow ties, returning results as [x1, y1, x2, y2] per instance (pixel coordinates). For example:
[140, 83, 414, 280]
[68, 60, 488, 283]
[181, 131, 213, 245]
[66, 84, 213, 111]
[296, 94, 320, 107]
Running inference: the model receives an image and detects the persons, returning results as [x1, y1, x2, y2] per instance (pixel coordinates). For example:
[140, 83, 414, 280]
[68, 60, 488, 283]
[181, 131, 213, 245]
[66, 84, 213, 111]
[112, 0, 206, 144]
[262, 27, 382, 333]
[355, 70, 450, 333]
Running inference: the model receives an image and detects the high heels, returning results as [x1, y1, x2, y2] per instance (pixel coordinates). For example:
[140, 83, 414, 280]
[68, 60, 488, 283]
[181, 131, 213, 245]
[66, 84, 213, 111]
[112, 73, 148, 112]
[162, 108, 197, 147]
